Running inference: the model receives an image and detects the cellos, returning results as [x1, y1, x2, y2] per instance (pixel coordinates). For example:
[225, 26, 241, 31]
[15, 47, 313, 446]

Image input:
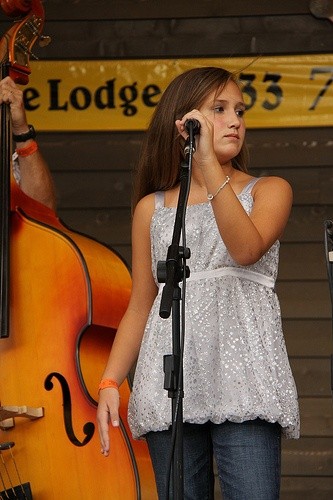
[1, 0, 161, 500]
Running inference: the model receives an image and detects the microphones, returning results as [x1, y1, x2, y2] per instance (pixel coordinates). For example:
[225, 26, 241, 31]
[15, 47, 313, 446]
[184, 118, 201, 135]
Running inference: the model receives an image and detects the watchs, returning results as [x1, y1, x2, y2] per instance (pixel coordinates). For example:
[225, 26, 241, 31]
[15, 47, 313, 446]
[12, 124, 36, 143]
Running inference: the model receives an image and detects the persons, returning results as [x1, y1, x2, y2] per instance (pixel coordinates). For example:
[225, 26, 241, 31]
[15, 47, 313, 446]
[0, 76, 56, 212]
[96, 67, 301, 499]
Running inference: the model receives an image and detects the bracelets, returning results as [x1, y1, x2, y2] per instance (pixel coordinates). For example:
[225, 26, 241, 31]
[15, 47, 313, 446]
[17, 140, 38, 157]
[98, 380, 119, 393]
[207, 176, 230, 200]
